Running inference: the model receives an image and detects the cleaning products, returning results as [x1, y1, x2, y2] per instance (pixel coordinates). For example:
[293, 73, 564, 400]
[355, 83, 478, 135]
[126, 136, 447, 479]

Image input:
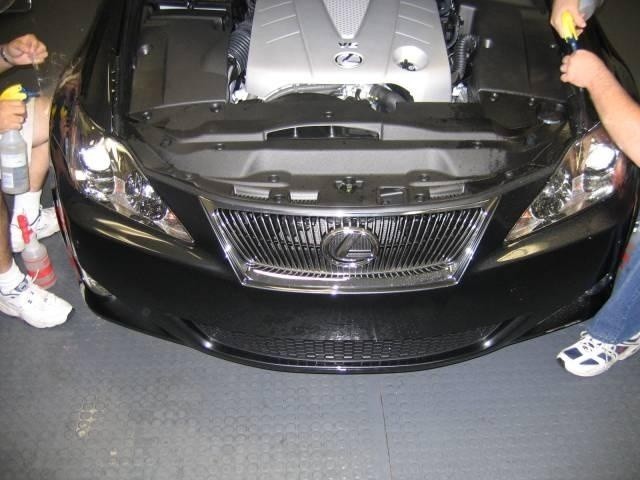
[0, 83, 41, 196]
[561, 0, 605, 57]
[17, 215, 56, 290]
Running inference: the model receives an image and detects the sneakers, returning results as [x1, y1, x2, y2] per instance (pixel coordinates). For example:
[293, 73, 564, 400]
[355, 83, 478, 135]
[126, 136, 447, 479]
[0, 270, 73, 328]
[556, 331, 640, 377]
[9, 207, 61, 253]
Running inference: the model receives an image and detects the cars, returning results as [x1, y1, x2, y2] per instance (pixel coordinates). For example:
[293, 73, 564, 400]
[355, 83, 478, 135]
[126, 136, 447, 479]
[47, 2, 640, 376]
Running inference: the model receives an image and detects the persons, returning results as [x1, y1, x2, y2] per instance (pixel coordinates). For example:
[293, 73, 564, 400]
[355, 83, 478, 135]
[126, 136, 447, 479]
[0, 33, 74, 330]
[550, 0, 640, 376]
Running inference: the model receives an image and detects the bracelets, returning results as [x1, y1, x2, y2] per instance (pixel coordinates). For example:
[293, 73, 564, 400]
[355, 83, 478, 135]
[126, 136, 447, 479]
[1, 44, 16, 67]
